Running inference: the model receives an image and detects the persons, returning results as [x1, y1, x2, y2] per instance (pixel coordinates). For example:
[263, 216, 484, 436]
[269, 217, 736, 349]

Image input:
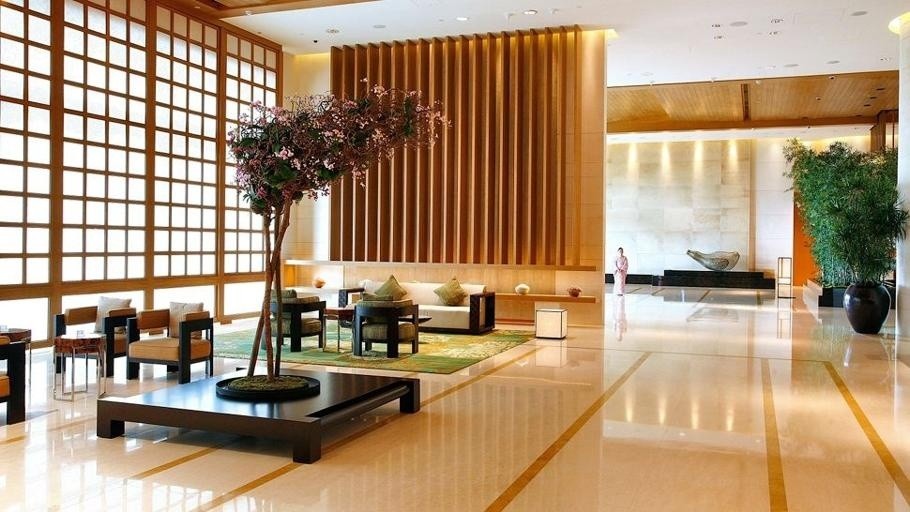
[613, 247, 629, 296]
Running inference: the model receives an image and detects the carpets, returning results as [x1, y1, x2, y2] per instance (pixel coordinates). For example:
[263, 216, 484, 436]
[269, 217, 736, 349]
[198, 328, 536, 373]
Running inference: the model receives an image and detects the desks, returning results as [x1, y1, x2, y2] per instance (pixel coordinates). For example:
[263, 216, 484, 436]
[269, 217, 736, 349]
[1, 329, 32, 389]
[55, 333, 107, 401]
[98, 366, 419, 463]
[323, 306, 358, 352]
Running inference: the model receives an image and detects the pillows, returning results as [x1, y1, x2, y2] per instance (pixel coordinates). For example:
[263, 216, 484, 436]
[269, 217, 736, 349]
[362, 295, 396, 324]
[95, 297, 131, 335]
[269, 290, 301, 320]
[169, 303, 202, 339]
[373, 276, 405, 301]
[434, 276, 468, 306]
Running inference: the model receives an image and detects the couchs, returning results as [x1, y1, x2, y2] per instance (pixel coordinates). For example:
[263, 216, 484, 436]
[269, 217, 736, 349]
[339, 275, 495, 334]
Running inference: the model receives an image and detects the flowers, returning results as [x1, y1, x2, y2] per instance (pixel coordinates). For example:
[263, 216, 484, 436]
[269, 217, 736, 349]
[223, 75, 452, 378]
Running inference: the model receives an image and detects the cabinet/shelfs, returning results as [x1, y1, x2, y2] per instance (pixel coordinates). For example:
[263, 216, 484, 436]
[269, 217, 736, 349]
[495, 293, 595, 303]
[282, 261, 345, 320]
[652, 270, 775, 289]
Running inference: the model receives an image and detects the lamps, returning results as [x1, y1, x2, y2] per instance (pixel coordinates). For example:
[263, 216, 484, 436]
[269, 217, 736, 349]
[535, 308, 568, 339]
[776, 257, 792, 286]
[536, 339, 569, 368]
[778, 282, 791, 299]
[887, 11, 910, 37]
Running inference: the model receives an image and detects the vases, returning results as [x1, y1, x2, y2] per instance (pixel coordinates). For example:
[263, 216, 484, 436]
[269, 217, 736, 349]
[843, 333, 891, 386]
[515, 284, 529, 295]
[215, 374, 320, 403]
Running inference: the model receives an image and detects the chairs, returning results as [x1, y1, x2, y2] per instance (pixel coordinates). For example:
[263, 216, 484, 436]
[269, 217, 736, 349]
[53, 296, 135, 379]
[1, 339, 27, 425]
[259, 290, 327, 352]
[127, 302, 213, 384]
[354, 293, 419, 359]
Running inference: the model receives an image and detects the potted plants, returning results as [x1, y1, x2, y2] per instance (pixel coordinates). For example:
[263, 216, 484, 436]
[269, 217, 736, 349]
[782, 137, 909, 335]
